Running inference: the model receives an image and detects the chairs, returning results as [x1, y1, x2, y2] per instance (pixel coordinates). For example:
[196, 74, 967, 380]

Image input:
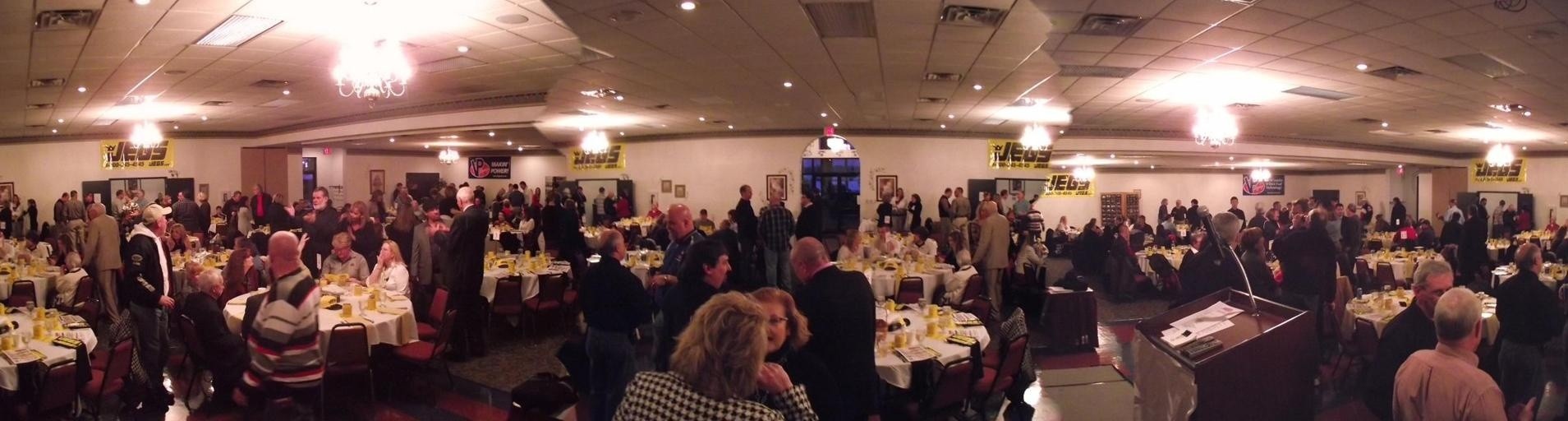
[0, 217, 1567, 421]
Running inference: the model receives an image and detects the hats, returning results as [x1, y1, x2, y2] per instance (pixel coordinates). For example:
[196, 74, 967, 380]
[141, 204, 172, 226]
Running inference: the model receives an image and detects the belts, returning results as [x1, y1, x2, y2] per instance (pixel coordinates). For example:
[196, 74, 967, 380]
[68, 217, 82, 220]
[955, 215, 968, 218]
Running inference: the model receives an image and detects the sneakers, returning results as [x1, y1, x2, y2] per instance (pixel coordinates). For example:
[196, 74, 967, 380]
[144, 386, 175, 406]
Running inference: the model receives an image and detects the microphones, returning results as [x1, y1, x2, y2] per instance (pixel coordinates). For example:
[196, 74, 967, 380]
[1197, 205, 1225, 260]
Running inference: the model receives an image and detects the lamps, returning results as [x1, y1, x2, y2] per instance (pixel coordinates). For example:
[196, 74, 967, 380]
[128, 121, 164, 151]
[437, 148, 459, 164]
[581, 129, 610, 154]
[1251, 168, 1271, 183]
[1072, 165, 1096, 183]
[1191, 102, 1238, 149]
[331, 29, 417, 105]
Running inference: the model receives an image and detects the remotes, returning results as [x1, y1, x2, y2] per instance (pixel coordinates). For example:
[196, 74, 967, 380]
[1179, 336, 1214, 352]
[1186, 339, 1222, 360]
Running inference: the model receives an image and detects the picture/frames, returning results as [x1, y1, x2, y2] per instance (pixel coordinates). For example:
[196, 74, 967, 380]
[199, 184, 209, 198]
[675, 185, 685, 198]
[0, 182, 14, 208]
[876, 176, 898, 202]
[766, 174, 787, 200]
[370, 169, 385, 194]
[1355, 191, 1366, 209]
[1560, 195, 1568, 207]
[662, 181, 672, 193]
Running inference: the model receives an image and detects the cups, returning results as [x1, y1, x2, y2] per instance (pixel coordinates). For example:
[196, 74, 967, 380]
[847, 231, 954, 357]
[482, 217, 663, 274]
[169, 246, 408, 329]
[0, 240, 66, 353]
[1145, 244, 1192, 257]
[1353, 227, 1566, 315]
